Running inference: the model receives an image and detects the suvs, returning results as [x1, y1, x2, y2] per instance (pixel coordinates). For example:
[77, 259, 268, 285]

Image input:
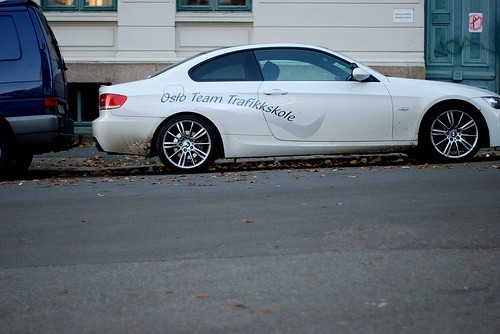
[0, 0, 83, 179]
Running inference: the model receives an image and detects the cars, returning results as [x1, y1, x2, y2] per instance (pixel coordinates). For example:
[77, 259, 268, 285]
[91, 43, 498, 175]
